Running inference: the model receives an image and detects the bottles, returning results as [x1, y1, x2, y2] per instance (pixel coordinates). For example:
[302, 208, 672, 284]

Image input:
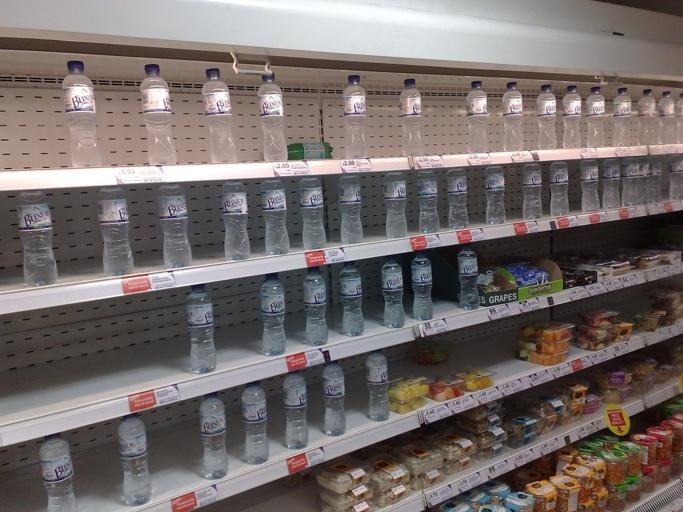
[637, 88, 682, 145]
[466, 81, 490, 153]
[61, 59, 105, 168]
[320, 362, 347, 437]
[339, 261, 366, 337]
[94, 185, 137, 275]
[288, 140, 333, 159]
[137, 61, 178, 167]
[257, 74, 288, 162]
[400, 79, 425, 157]
[563, 85, 582, 149]
[537, 84, 557, 150]
[338, 173, 363, 244]
[382, 255, 405, 328]
[343, 75, 369, 159]
[580, 158, 601, 213]
[416, 170, 440, 234]
[446, 167, 469, 229]
[602, 154, 683, 210]
[501, 82, 524, 152]
[303, 265, 329, 347]
[185, 283, 218, 377]
[15, 189, 60, 288]
[613, 87, 632, 146]
[201, 66, 239, 166]
[156, 182, 193, 270]
[411, 249, 434, 322]
[221, 179, 251, 262]
[196, 389, 228, 481]
[260, 179, 290, 255]
[366, 349, 390, 422]
[257, 273, 286, 356]
[383, 170, 408, 238]
[458, 243, 480, 310]
[586, 86, 605, 147]
[484, 164, 506, 225]
[550, 161, 569, 216]
[37, 432, 77, 512]
[240, 382, 269, 463]
[299, 176, 327, 250]
[116, 412, 153, 506]
[281, 370, 311, 450]
[522, 162, 541, 220]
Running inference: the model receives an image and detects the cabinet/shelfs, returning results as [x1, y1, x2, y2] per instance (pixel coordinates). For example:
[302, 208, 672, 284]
[0, 0, 683, 512]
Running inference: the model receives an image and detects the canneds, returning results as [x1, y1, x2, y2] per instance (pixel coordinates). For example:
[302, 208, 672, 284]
[439, 396, 683, 512]
